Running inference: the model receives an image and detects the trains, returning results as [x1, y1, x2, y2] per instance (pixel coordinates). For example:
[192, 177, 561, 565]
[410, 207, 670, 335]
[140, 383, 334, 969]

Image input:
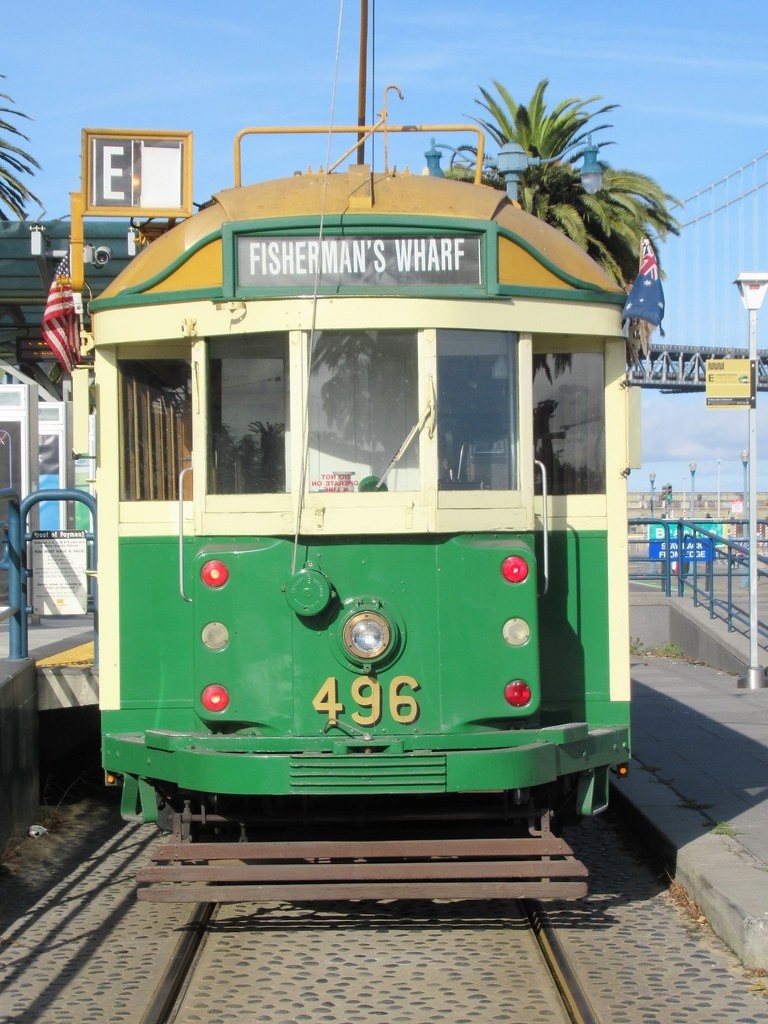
[62, 86, 655, 907]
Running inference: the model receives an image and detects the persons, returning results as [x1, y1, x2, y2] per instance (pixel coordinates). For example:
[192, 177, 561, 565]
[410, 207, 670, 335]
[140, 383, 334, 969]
[731, 536, 748, 568]
[705, 512, 712, 518]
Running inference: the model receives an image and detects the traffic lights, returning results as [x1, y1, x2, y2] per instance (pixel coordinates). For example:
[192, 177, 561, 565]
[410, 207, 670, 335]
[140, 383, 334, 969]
[666, 485, 673, 500]
[662, 486, 668, 500]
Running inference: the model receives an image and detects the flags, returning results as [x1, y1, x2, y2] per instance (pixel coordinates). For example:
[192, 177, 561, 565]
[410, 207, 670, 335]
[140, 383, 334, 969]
[41, 251, 81, 376]
[621, 237, 666, 338]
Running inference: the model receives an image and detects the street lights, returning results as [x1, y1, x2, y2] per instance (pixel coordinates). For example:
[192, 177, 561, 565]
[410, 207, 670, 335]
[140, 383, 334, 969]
[688, 457, 698, 518]
[734, 272, 768, 692]
[650, 472, 656, 519]
[740, 446, 749, 539]
